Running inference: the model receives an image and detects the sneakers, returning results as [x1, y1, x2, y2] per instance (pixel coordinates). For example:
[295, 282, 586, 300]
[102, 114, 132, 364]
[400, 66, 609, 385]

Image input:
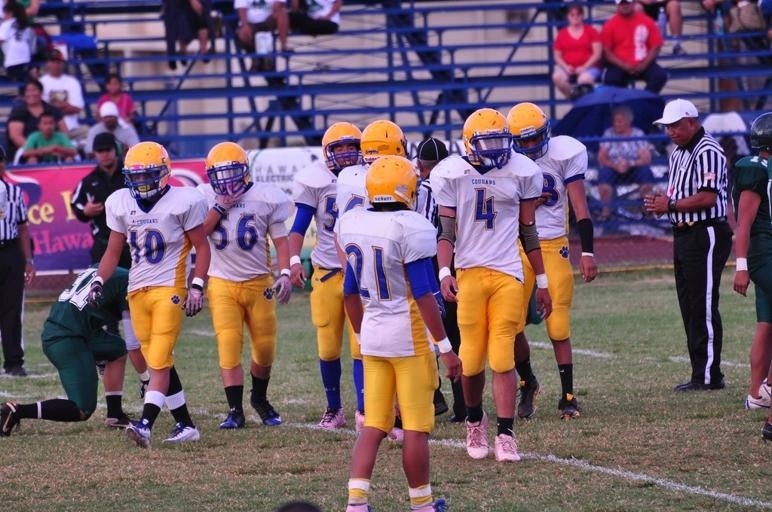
[674, 376, 726, 392]
[433, 498, 449, 512]
[672, 44, 687, 57]
[745, 377, 771, 440]
[5, 366, 27, 376]
[0, 401, 18, 438]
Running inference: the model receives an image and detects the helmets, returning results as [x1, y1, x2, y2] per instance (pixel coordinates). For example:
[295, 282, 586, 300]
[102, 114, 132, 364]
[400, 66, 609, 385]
[322, 119, 418, 210]
[205, 141, 250, 196]
[750, 112, 771, 150]
[507, 102, 551, 161]
[462, 108, 512, 169]
[122, 141, 172, 202]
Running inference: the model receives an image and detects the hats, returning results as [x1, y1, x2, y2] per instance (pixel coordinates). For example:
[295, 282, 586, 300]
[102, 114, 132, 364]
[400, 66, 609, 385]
[93, 133, 115, 151]
[100, 101, 119, 118]
[652, 98, 699, 125]
[47, 48, 66, 64]
[416, 137, 449, 161]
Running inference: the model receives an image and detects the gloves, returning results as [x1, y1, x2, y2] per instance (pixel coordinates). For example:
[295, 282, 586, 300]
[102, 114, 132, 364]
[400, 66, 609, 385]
[214, 184, 246, 214]
[271, 269, 292, 304]
[139, 370, 150, 399]
[181, 277, 204, 317]
[88, 277, 104, 307]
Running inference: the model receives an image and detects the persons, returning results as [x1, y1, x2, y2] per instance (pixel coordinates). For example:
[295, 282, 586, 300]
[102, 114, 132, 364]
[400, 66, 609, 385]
[331, 153, 464, 512]
[2, 258, 155, 438]
[643, 97, 772, 441]
[73, 127, 296, 452]
[553, 0, 772, 227]
[284, 101, 596, 440]
[427, 106, 553, 463]
[155, 0, 342, 74]
[0, 1, 145, 174]
[0, 181, 36, 376]
